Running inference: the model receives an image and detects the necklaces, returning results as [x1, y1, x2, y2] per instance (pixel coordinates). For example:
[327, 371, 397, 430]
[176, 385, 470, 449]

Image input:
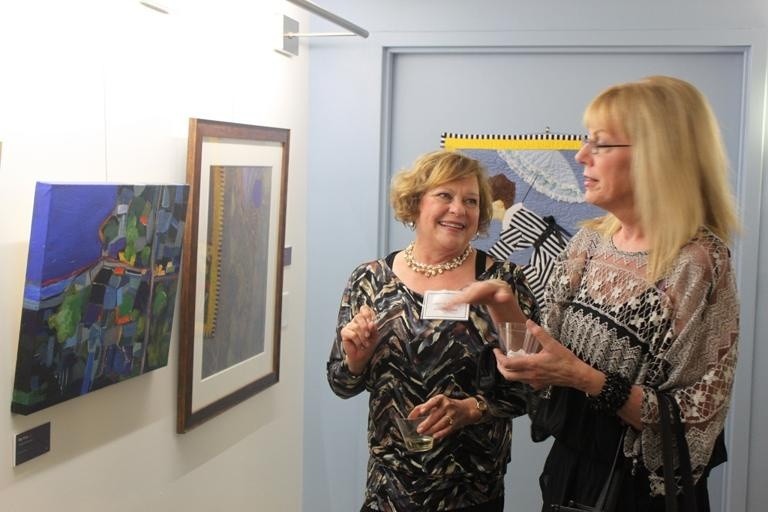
[404, 241, 472, 276]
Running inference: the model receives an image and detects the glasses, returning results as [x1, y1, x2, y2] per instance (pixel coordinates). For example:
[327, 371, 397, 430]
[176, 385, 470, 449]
[579, 135, 630, 155]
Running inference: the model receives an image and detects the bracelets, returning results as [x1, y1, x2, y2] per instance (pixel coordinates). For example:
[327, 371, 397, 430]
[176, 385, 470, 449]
[589, 372, 633, 412]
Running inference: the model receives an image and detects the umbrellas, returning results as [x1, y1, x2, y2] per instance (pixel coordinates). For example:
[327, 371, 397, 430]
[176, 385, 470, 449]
[497, 150, 585, 209]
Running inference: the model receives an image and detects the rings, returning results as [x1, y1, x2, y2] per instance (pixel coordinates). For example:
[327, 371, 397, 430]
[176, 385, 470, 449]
[445, 415, 455, 426]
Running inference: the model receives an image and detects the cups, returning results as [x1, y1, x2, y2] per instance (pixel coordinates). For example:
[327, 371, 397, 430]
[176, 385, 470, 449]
[497, 319, 542, 359]
[393, 408, 435, 451]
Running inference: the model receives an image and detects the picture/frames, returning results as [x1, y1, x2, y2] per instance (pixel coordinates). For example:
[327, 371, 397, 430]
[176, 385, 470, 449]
[176, 118, 290, 434]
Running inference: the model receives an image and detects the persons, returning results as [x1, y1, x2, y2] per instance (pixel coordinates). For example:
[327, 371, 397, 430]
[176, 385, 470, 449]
[449, 75, 741, 512]
[326, 154, 542, 512]
[485, 174, 571, 305]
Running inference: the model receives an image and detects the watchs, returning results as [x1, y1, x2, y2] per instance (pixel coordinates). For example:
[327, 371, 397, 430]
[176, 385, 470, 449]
[472, 393, 488, 421]
